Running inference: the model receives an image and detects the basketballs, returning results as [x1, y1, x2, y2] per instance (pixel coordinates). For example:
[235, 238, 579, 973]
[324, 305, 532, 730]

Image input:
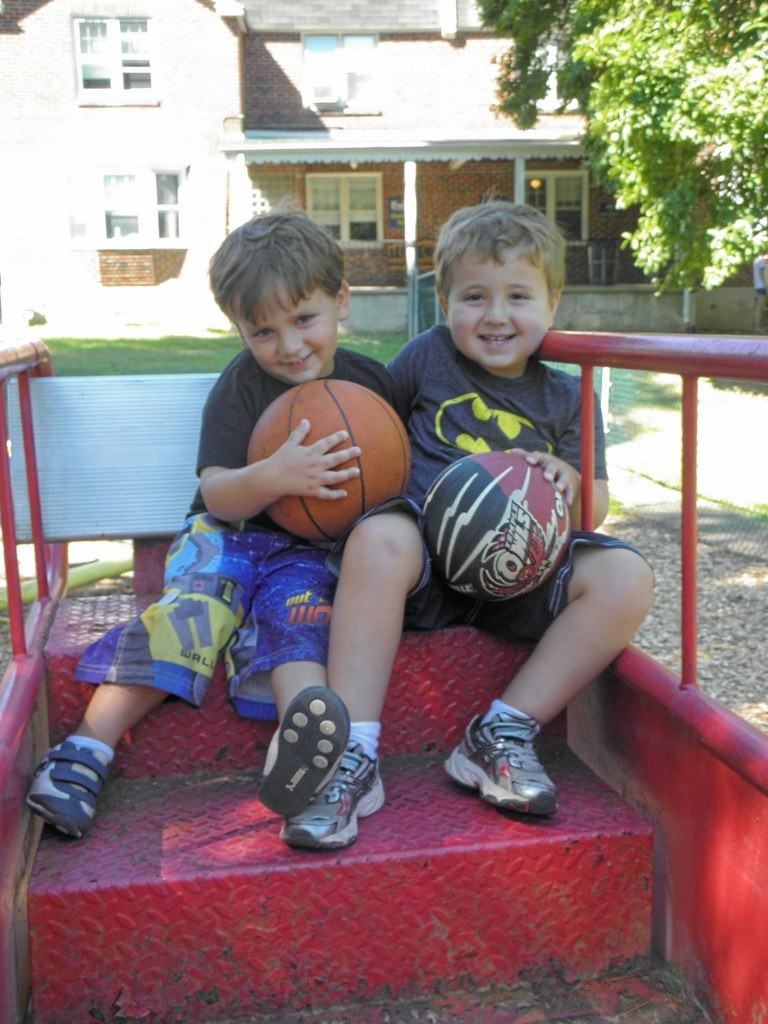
[420, 449, 568, 599]
[246, 378, 411, 543]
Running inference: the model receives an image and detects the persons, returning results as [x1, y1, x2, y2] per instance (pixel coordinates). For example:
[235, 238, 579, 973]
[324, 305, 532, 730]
[752, 249, 768, 333]
[280, 202, 654, 851]
[26, 210, 410, 839]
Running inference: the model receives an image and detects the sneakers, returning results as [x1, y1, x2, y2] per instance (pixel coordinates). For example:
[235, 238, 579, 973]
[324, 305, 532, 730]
[445, 711, 557, 814]
[279, 739, 385, 849]
[25, 735, 114, 838]
[258, 686, 350, 818]
